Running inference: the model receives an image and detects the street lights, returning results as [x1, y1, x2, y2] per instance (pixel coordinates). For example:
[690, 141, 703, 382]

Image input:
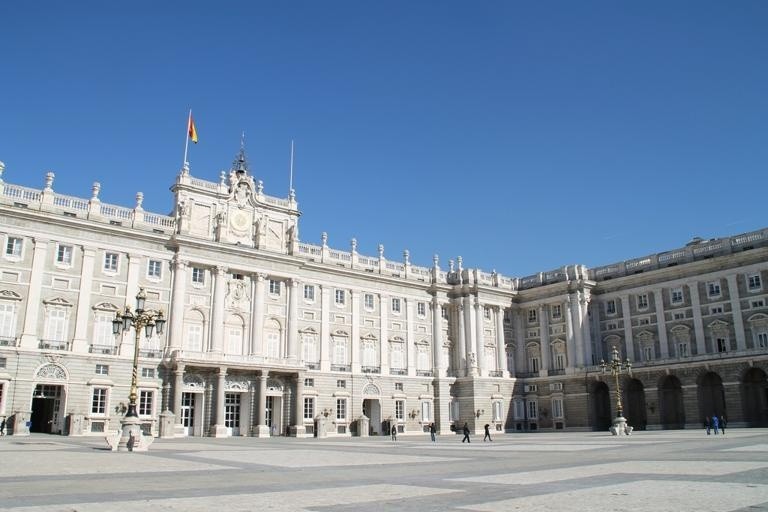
[599, 345, 632, 417]
[112, 285, 166, 417]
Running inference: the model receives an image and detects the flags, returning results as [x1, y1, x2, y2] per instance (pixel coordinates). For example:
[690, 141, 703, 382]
[187, 115, 198, 144]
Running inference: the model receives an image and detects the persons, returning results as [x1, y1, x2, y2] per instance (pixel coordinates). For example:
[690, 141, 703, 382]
[0, 418, 6, 437]
[704, 416, 711, 435]
[390, 425, 397, 442]
[483, 424, 491, 441]
[711, 413, 719, 434]
[428, 423, 435, 444]
[718, 416, 726, 435]
[460, 421, 470, 443]
